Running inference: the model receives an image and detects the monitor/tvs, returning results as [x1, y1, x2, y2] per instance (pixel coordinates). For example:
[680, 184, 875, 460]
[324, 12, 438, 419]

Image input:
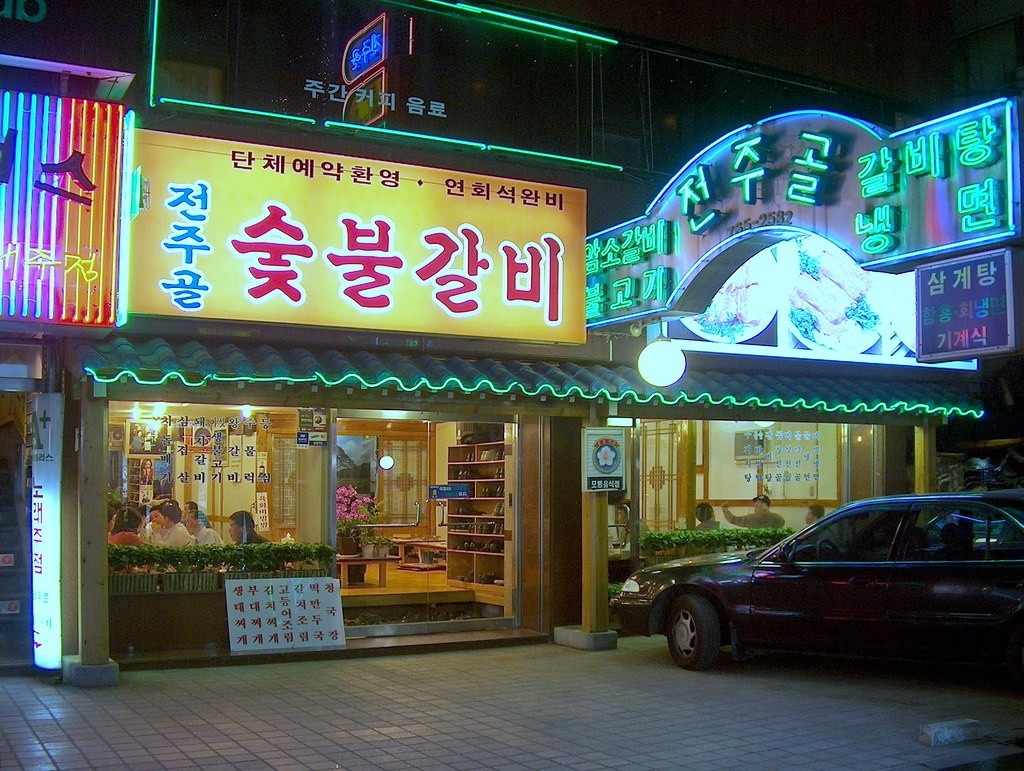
[734, 428, 768, 459]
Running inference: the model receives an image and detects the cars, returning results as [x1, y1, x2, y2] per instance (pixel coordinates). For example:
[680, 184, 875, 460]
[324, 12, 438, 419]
[608, 490, 1023, 675]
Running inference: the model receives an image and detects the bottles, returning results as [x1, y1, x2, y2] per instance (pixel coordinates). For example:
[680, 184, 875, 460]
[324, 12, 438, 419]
[451, 448, 505, 585]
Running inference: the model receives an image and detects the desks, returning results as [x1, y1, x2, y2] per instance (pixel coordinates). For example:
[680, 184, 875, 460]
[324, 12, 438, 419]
[337, 554, 403, 589]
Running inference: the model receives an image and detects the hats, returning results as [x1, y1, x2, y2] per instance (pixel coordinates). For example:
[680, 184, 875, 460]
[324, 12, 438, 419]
[752, 495, 770, 506]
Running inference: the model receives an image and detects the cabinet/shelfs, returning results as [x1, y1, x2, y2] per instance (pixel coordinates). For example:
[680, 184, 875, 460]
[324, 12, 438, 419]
[446, 439, 505, 593]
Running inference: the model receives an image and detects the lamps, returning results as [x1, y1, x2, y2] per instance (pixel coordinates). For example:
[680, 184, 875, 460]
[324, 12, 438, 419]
[628, 314, 686, 386]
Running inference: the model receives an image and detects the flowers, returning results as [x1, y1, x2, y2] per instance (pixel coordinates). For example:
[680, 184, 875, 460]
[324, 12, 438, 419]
[336, 485, 380, 538]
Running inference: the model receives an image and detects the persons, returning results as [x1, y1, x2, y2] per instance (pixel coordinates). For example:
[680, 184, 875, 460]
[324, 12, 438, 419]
[108, 499, 193, 546]
[805, 505, 824, 523]
[721, 494, 786, 529]
[229, 510, 269, 546]
[186, 510, 224, 546]
[151, 492, 166, 507]
[696, 502, 727, 529]
[184, 501, 211, 528]
[619, 504, 630, 524]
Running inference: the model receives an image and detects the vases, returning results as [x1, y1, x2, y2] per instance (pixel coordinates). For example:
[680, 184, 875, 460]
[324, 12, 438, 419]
[337, 534, 361, 559]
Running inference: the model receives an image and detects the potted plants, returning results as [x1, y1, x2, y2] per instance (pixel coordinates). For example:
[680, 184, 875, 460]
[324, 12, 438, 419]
[637, 529, 794, 558]
[106, 544, 159, 593]
[158, 544, 225, 593]
[224, 542, 285, 582]
[375, 537, 392, 558]
[281, 541, 334, 576]
[360, 527, 378, 557]
